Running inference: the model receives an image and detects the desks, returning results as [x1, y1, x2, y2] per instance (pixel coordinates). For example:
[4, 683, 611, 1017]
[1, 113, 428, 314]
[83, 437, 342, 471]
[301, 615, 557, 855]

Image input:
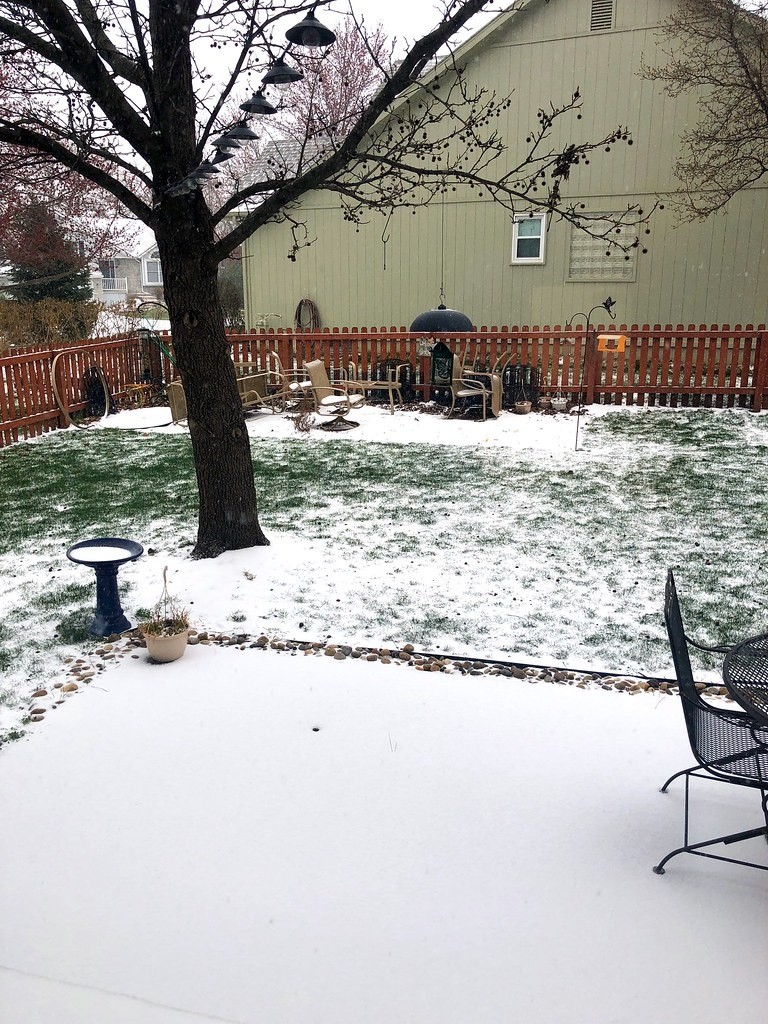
[722, 632, 768, 728]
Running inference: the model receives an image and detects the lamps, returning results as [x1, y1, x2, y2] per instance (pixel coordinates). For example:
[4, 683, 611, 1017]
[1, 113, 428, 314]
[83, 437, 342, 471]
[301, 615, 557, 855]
[409, 176, 474, 332]
[157, 1, 337, 199]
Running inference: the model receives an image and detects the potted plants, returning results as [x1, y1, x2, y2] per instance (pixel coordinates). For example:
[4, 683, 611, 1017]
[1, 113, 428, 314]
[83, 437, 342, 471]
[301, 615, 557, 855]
[538, 376, 552, 409]
[550, 376, 568, 411]
[514, 378, 532, 414]
[136, 565, 191, 663]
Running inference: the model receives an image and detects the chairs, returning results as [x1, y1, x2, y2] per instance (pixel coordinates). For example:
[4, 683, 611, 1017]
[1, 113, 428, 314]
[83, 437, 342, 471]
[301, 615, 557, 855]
[459, 341, 517, 419]
[652, 567, 768, 875]
[444, 352, 490, 422]
[165, 358, 409, 434]
